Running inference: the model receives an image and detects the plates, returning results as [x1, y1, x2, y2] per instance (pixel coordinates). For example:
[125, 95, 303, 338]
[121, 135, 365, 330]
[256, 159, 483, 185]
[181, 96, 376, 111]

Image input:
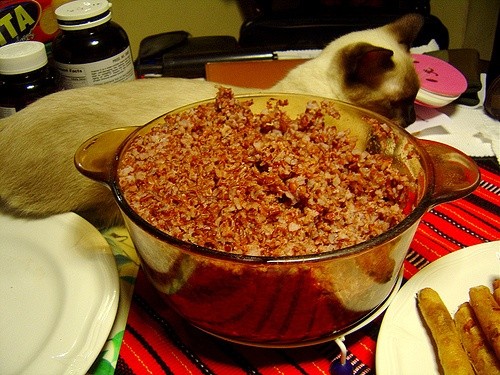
[0, 209, 119, 375]
[375, 236, 500, 374]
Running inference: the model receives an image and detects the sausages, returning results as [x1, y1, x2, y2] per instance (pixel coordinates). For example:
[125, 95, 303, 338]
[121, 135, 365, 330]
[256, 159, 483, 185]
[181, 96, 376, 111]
[454, 301, 500, 375]
[467, 285, 500, 359]
[417, 287, 474, 375]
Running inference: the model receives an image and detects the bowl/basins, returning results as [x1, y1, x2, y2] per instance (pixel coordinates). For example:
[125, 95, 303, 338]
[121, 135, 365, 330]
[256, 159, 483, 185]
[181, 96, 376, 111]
[74, 96, 481, 340]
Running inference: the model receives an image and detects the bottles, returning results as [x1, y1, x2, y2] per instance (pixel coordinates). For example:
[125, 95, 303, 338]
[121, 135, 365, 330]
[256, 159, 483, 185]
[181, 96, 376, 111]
[1, 40, 59, 117]
[50, 2, 135, 81]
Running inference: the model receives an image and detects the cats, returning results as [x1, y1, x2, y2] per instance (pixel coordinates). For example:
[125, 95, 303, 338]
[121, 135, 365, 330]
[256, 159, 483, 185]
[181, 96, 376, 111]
[0, 14, 425, 233]
